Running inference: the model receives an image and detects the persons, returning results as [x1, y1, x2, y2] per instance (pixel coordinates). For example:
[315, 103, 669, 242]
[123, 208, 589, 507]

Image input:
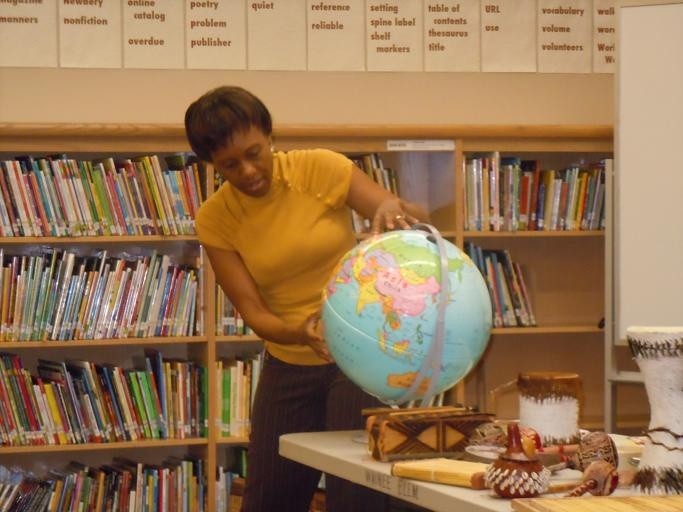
[186, 86, 430, 512]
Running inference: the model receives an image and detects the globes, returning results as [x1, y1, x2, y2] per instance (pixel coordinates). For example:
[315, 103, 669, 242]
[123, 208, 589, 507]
[321, 223, 495, 408]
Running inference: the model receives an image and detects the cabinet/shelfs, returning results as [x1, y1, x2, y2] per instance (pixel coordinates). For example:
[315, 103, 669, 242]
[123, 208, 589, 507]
[0, 121, 615, 512]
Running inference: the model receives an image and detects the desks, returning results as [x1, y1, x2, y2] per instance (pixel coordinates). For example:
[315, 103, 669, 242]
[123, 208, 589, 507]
[277, 415, 683, 512]
